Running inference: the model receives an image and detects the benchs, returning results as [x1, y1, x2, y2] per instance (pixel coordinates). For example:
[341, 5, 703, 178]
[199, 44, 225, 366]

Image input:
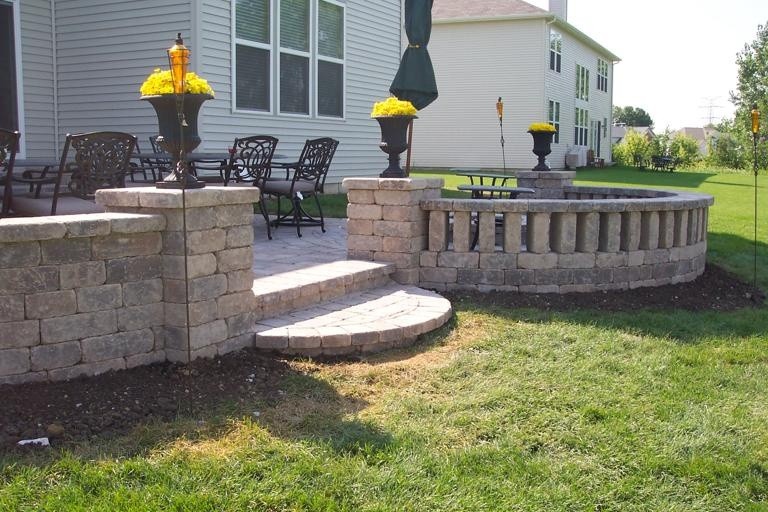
[455, 182, 535, 200]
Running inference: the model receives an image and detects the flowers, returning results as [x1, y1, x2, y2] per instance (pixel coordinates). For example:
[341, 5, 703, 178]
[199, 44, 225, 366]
[369, 96, 416, 116]
[527, 122, 555, 131]
[138, 66, 215, 96]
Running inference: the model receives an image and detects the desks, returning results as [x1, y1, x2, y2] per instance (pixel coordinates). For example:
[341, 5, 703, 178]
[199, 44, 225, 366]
[130, 148, 288, 229]
[1, 157, 67, 198]
[455, 169, 515, 199]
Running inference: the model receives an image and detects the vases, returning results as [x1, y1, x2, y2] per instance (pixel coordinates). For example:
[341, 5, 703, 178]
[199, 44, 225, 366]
[139, 93, 213, 189]
[527, 130, 555, 171]
[371, 114, 418, 177]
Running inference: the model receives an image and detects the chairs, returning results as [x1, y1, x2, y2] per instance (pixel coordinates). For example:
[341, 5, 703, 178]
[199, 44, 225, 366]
[0, 128, 21, 217]
[146, 134, 198, 181]
[203, 136, 279, 240]
[116, 138, 156, 187]
[257, 137, 338, 237]
[8, 130, 138, 215]
[630, 151, 680, 172]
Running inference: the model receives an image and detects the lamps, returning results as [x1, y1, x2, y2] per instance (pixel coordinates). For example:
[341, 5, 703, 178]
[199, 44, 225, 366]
[167, 31, 190, 160]
[495, 95, 506, 175]
[750, 99, 759, 298]
